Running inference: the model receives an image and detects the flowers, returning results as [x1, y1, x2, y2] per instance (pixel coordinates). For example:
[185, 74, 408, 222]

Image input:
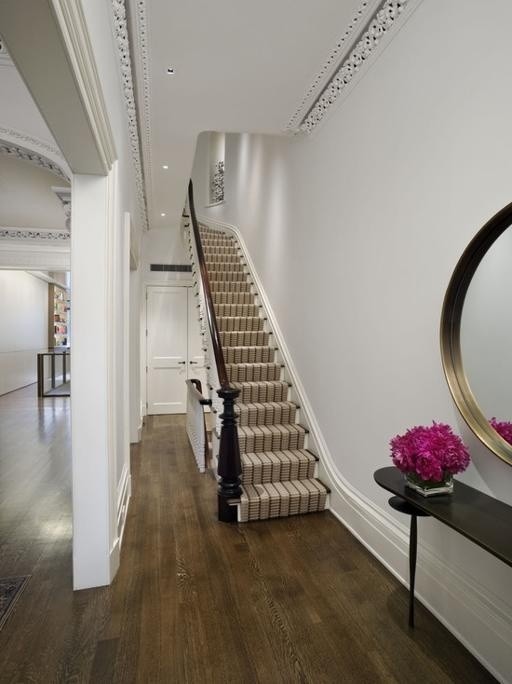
[489, 416, 511, 446]
[387, 420, 471, 483]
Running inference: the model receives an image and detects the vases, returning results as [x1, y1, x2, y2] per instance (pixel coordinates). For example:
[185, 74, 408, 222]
[403, 469, 454, 497]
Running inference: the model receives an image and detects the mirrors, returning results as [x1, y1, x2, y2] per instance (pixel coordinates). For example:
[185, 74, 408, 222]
[439, 201, 512, 467]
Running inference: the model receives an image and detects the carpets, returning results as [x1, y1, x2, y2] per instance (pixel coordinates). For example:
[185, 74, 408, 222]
[1, 574, 32, 633]
[197, 219, 327, 523]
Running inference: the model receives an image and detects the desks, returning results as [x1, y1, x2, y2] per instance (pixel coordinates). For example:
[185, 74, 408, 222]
[373, 466, 512, 627]
